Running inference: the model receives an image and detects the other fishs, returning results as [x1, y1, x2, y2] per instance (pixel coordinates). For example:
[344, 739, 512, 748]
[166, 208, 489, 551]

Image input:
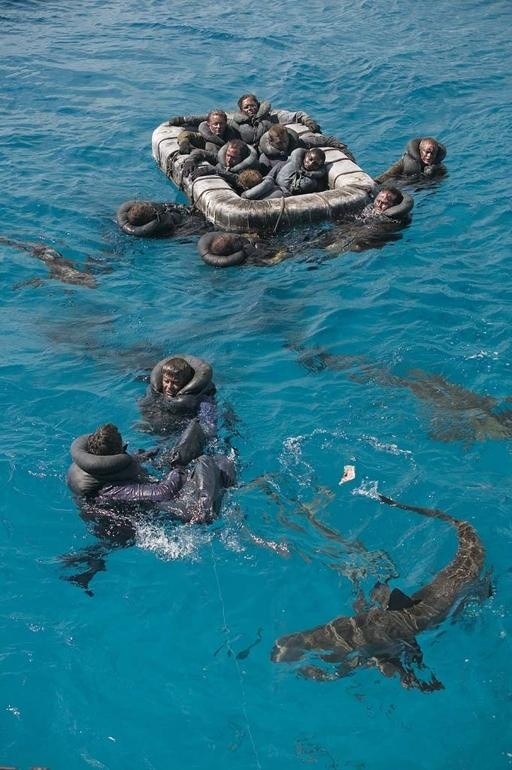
[2, 232, 108, 293]
[267, 494, 486, 693]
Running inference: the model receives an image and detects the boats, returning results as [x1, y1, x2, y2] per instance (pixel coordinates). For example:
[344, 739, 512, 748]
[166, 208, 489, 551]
[152, 110, 380, 236]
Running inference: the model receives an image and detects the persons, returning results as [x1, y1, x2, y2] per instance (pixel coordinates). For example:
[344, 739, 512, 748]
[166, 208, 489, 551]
[266, 147, 331, 193]
[131, 354, 218, 441]
[169, 94, 321, 145]
[185, 135, 261, 192]
[196, 226, 294, 269]
[306, 184, 414, 253]
[236, 169, 292, 198]
[116, 200, 215, 236]
[372, 137, 448, 189]
[255, 123, 363, 180]
[64, 418, 180, 522]
[178, 108, 244, 156]
